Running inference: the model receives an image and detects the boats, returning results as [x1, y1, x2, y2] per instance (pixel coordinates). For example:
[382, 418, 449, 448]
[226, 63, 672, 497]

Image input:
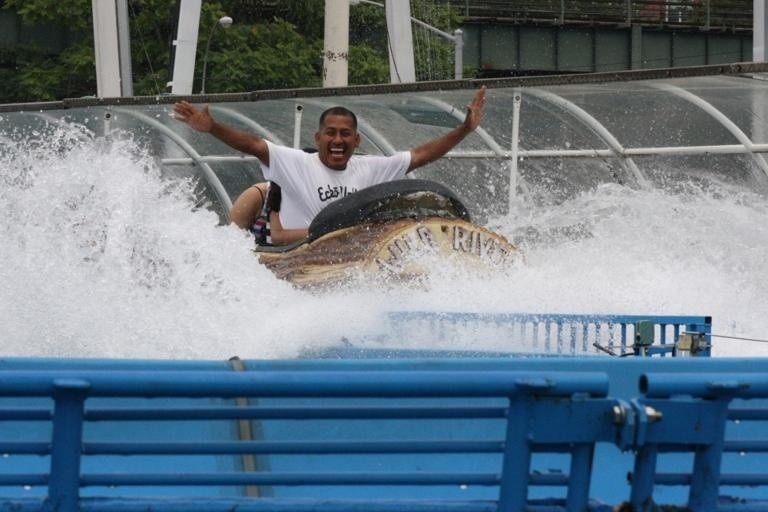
[229, 182, 524, 303]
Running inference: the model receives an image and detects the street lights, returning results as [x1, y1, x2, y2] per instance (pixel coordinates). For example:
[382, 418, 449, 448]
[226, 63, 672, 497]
[201, 15, 233, 95]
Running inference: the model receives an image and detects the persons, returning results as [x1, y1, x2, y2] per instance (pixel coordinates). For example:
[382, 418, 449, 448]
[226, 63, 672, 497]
[172, 84, 490, 230]
[265, 147, 318, 246]
[228, 181, 270, 244]
